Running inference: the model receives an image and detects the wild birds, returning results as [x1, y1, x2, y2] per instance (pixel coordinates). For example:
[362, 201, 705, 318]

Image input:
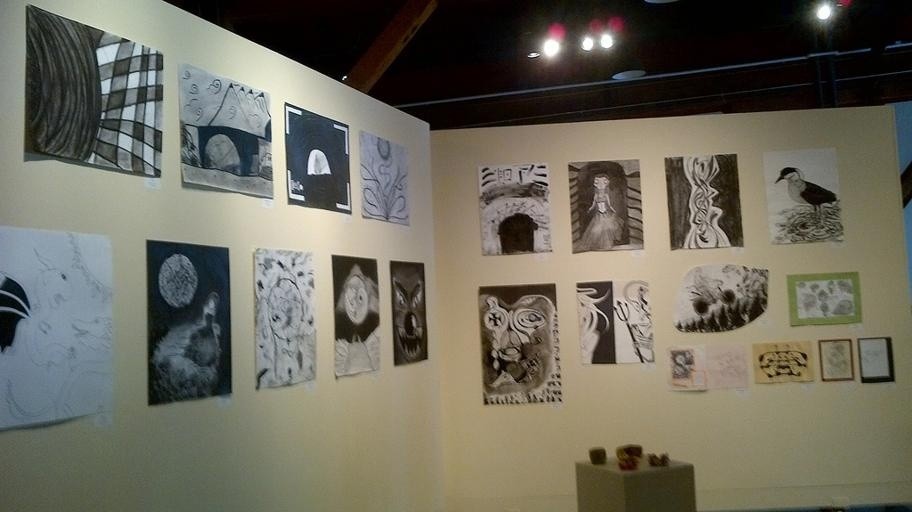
[773, 167, 838, 215]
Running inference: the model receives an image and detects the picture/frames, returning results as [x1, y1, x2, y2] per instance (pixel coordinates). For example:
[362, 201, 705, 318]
[786, 270, 863, 326]
